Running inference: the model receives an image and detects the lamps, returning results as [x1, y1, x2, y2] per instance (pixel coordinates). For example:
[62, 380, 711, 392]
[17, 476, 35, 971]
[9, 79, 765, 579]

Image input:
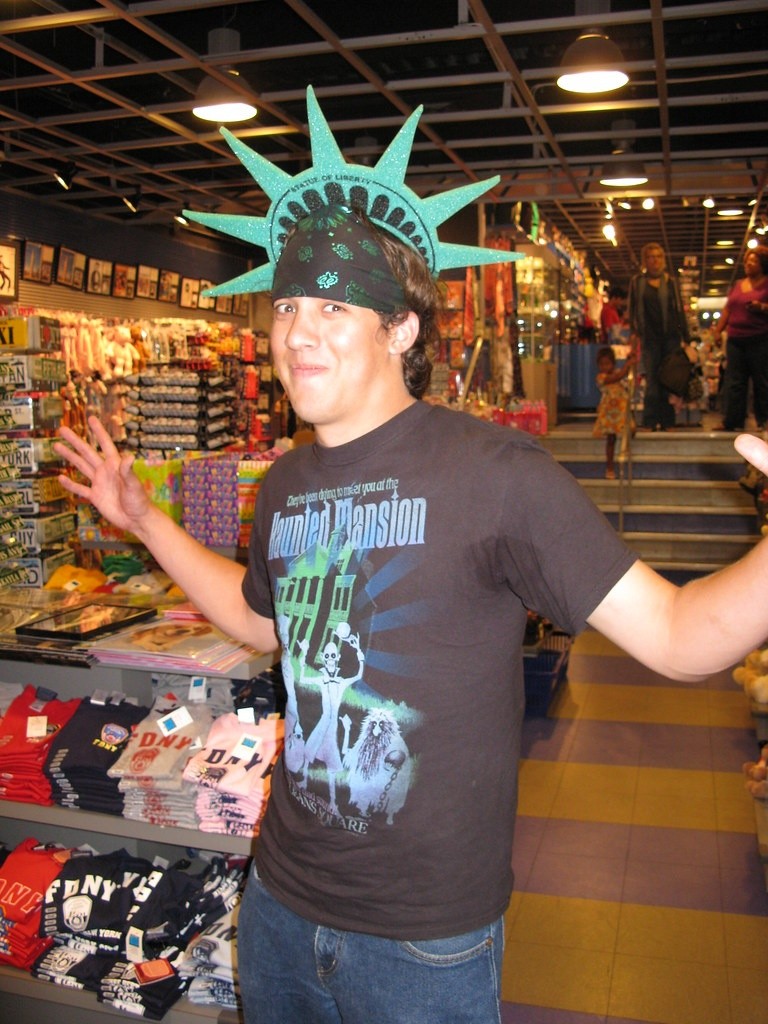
[557, 3, 632, 93]
[600, 122, 768, 319]
[49, 166, 192, 230]
[193, 28, 257, 121]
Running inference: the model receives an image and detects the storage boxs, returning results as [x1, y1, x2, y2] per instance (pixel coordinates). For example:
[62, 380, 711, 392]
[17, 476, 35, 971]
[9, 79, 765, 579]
[1, 331, 551, 585]
[432, 281, 467, 311]
[432, 307, 465, 339]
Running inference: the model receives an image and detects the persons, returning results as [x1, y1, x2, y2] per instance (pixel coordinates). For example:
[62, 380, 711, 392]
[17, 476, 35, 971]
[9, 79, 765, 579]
[627, 242, 692, 432]
[591, 347, 639, 482]
[49, 204, 767, 1024]
[711, 246, 768, 431]
[601, 287, 630, 344]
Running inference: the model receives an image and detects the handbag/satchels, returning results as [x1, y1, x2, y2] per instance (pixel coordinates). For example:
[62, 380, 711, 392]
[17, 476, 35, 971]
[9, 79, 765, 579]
[654, 346, 696, 393]
[681, 344, 704, 401]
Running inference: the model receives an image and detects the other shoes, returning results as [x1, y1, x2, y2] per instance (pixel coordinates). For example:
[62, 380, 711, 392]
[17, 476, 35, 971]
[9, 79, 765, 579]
[711, 423, 745, 431]
[605, 468, 615, 478]
[756, 422, 765, 431]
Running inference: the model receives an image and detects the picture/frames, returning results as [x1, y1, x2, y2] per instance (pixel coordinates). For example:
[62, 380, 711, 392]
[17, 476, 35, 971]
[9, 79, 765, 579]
[0, 237, 269, 317]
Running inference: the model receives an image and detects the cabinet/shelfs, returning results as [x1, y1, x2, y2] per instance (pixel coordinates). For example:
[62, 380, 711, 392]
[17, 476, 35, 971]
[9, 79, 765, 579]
[0, 794, 260, 1023]
